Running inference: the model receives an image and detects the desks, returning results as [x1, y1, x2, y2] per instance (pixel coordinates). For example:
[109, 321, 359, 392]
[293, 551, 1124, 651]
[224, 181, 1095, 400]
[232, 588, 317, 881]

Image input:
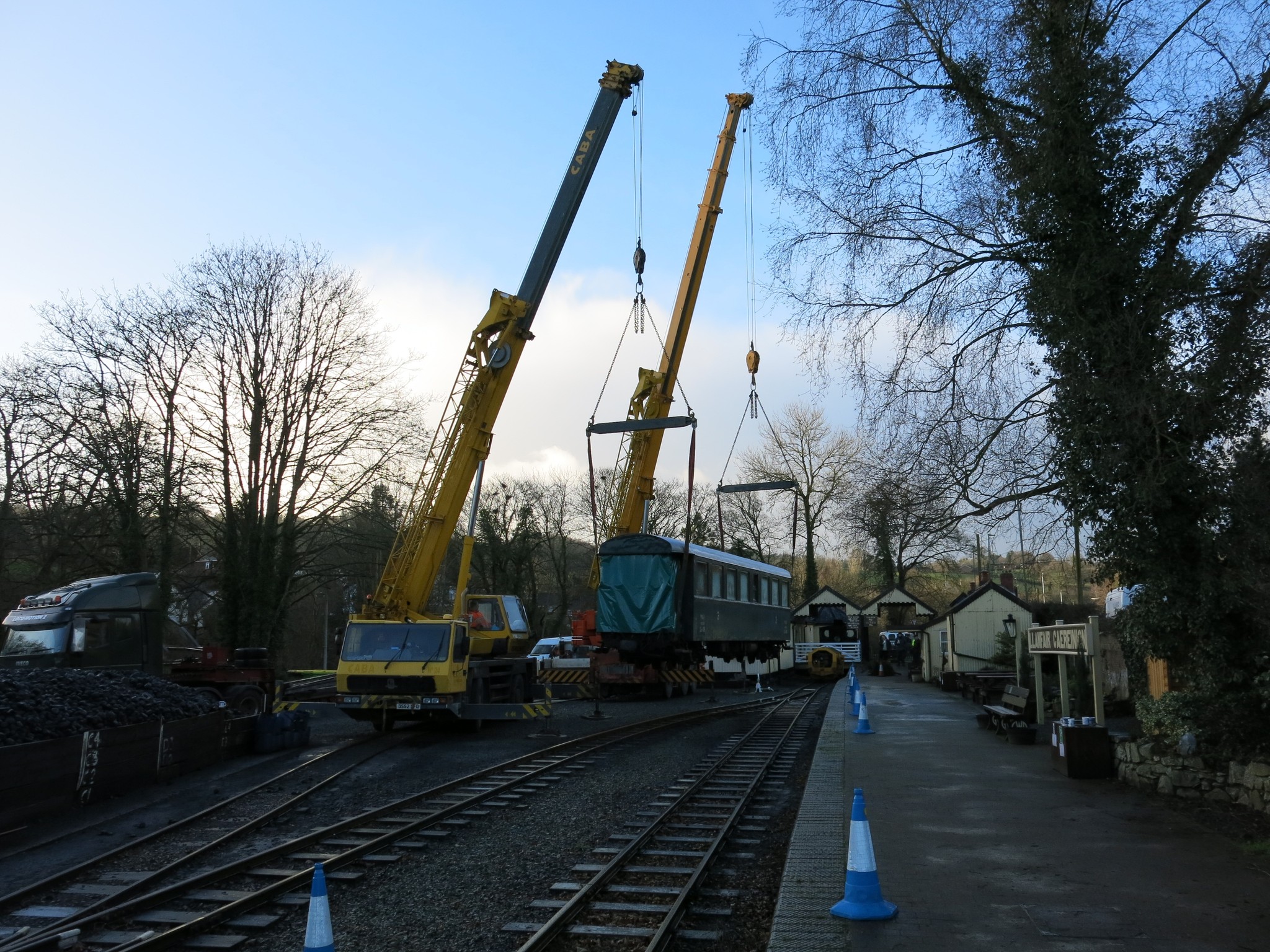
[977, 675, 1016, 681]
[965, 673, 1017, 676]
[1051, 721, 1112, 778]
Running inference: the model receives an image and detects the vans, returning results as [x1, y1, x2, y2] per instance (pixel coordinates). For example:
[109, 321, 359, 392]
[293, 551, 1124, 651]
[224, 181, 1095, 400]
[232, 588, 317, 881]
[1105, 584, 1168, 619]
[526, 636, 583, 661]
[0, 570, 203, 679]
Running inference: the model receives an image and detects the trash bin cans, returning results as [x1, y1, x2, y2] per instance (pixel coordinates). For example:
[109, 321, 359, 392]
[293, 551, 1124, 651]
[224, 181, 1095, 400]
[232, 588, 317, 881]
[940, 670, 965, 692]
[1050, 719, 1111, 779]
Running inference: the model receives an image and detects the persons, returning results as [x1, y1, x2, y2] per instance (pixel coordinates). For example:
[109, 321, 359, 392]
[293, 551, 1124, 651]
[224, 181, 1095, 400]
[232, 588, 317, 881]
[879, 635, 892, 662]
[904, 634, 922, 670]
[467, 600, 500, 630]
[894, 632, 906, 667]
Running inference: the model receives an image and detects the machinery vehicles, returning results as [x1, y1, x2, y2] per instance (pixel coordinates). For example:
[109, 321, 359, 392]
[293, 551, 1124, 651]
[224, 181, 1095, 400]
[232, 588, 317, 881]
[267, 57, 646, 758]
[539, 92, 755, 693]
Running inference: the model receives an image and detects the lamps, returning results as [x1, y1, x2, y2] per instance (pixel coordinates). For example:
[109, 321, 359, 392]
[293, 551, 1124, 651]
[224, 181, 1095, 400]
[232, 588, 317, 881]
[1002, 614, 1017, 638]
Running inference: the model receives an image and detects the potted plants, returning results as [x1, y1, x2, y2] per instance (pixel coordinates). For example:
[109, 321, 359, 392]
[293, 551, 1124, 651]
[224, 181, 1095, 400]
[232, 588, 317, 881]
[910, 670, 923, 682]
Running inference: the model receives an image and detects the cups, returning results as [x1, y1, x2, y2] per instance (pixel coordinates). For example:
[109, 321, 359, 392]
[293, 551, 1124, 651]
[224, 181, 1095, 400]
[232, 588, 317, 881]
[219, 701, 226, 707]
[1060, 717, 1070, 726]
[1068, 719, 1075, 727]
[1089, 717, 1096, 726]
[1082, 717, 1089, 725]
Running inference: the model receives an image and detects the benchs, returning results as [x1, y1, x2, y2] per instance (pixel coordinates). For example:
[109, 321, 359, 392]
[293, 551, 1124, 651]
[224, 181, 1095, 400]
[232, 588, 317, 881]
[982, 685, 1029, 741]
[960, 682, 1017, 705]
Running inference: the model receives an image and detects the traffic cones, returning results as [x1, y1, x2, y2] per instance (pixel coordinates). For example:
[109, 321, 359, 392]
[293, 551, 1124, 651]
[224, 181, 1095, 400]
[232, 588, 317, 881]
[878, 660, 886, 677]
[830, 788, 898, 922]
[851, 691, 876, 735]
[845, 662, 860, 703]
[302, 863, 336, 952]
[848, 683, 862, 716]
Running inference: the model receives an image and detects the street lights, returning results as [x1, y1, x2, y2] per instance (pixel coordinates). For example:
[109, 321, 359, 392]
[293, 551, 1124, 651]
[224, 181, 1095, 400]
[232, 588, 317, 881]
[988, 534, 996, 571]
[1013, 459, 1029, 602]
